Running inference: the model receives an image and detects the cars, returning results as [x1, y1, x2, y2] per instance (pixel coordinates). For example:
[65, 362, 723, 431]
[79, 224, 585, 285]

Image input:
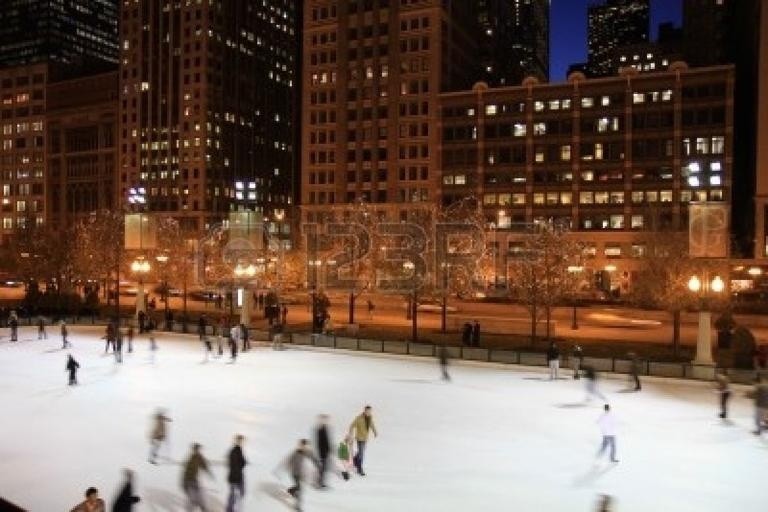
[113, 283, 225, 301]
[404, 297, 461, 312]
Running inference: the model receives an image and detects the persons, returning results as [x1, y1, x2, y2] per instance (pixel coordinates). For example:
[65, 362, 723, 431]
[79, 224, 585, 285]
[147, 410, 172, 464]
[8, 316, 18, 341]
[104, 291, 251, 362]
[314, 413, 331, 490]
[547, 342, 559, 380]
[472, 320, 481, 347]
[438, 343, 451, 381]
[461, 322, 473, 347]
[61, 322, 71, 348]
[225, 434, 248, 511]
[745, 374, 768, 435]
[65, 353, 80, 386]
[628, 353, 641, 389]
[338, 434, 353, 481]
[284, 437, 323, 510]
[182, 442, 216, 510]
[8, 311, 17, 320]
[572, 345, 584, 380]
[349, 407, 377, 476]
[37, 315, 48, 339]
[71, 487, 105, 511]
[265, 302, 289, 350]
[598, 404, 620, 463]
[367, 301, 374, 321]
[111, 468, 141, 511]
[713, 369, 730, 418]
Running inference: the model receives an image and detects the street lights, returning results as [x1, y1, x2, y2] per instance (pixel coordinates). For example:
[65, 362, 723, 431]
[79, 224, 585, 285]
[128, 184, 147, 316]
[687, 159, 722, 367]
[235, 180, 257, 324]
